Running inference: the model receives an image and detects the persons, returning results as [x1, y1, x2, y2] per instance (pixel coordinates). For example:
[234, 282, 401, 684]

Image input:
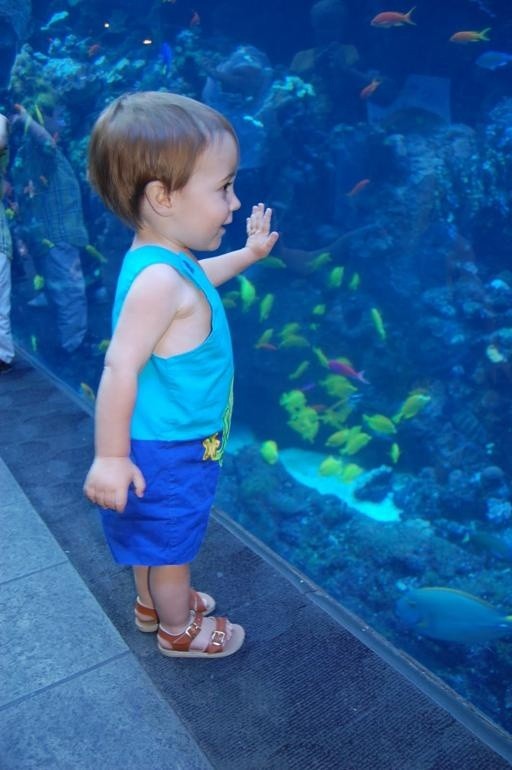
[11, 95, 91, 355]
[83, 90, 282, 660]
[0, 110, 18, 376]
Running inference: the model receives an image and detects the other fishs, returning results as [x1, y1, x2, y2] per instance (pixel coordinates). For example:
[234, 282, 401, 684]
[0, 0, 511, 483]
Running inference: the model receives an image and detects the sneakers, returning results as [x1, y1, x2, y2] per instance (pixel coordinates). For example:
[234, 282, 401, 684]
[54, 331, 110, 369]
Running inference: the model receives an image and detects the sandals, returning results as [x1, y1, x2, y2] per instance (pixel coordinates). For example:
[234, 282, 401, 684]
[154, 608, 246, 661]
[132, 590, 218, 634]
